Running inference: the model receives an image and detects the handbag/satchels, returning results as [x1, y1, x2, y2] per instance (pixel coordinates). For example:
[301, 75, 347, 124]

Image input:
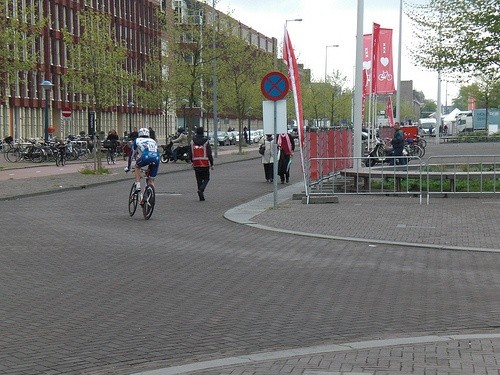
[259, 144, 265, 155]
[277, 147, 281, 160]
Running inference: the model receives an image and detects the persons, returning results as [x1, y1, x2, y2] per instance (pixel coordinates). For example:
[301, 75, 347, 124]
[148, 126, 155, 139]
[390, 121, 407, 166]
[190, 128, 214, 201]
[131, 127, 159, 205]
[277, 134, 295, 184]
[105, 128, 118, 160]
[171, 126, 190, 158]
[259, 134, 277, 183]
[130, 127, 138, 141]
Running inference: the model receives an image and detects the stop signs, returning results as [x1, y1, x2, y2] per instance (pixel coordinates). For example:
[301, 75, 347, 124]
[61, 107, 72, 117]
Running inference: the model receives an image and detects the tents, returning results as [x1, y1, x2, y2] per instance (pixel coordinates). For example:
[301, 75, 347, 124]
[444, 107, 467, 133]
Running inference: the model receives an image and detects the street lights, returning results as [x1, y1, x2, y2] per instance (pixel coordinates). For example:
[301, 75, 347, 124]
[127, 102, 136, 132]
[324, 44, 340, 126]
[285, 18, 303, 27]
[247, 107, 254, 145]
[39, 80, 54, 162]
[445, 80, 455, 114]
[180, 99, 190, 126]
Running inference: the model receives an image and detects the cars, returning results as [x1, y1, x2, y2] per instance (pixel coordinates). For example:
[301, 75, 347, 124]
[205, 128, 263, 146]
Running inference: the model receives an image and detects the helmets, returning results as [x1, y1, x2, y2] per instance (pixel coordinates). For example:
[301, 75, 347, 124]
[178, 127, 184, 133]
[138, 128, 150, 138]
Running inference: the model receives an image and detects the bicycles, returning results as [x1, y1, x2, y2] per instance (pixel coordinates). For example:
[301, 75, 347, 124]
[0, 130, 133, 167]
[362, 135, 428, 168]
[124, 162, 156, 221]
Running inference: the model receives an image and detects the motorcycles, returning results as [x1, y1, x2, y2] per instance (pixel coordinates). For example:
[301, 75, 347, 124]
[159, 139, 192, 164]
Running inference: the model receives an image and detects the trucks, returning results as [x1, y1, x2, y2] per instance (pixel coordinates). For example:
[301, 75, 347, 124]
[415, 108, 500, 136]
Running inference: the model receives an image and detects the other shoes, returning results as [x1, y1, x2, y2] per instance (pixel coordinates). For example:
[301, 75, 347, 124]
[197, 190, 205, 201]
[132, 188, 141, 195]
[281, 178, 284, 184]
[286, 177, 289, 182]
[267, 178, 274, 183]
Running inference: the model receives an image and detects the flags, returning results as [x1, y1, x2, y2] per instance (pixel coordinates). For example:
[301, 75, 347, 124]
[362, 23, 396, 95]
[283, 29, 304, 149]
[385, 96, 394, 126]
